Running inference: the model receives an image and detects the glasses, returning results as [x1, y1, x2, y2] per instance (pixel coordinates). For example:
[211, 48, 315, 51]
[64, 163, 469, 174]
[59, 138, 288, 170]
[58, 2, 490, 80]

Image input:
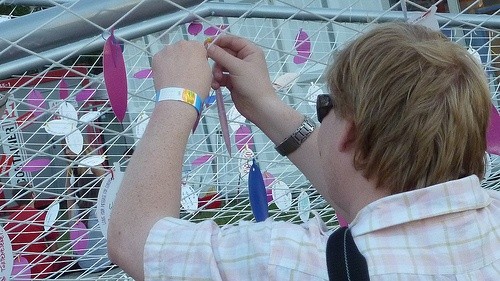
[316, 93, 333, 123]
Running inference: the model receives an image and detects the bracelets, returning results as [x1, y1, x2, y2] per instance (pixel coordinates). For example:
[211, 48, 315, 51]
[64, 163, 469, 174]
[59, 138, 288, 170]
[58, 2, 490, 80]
[274, 115, 316, 157]
[155, 87, 203, 115]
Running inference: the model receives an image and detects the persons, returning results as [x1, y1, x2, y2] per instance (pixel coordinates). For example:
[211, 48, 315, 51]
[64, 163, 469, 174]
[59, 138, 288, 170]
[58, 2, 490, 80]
[0, 135, 134, 281]
[106, 21, 500, 280]
